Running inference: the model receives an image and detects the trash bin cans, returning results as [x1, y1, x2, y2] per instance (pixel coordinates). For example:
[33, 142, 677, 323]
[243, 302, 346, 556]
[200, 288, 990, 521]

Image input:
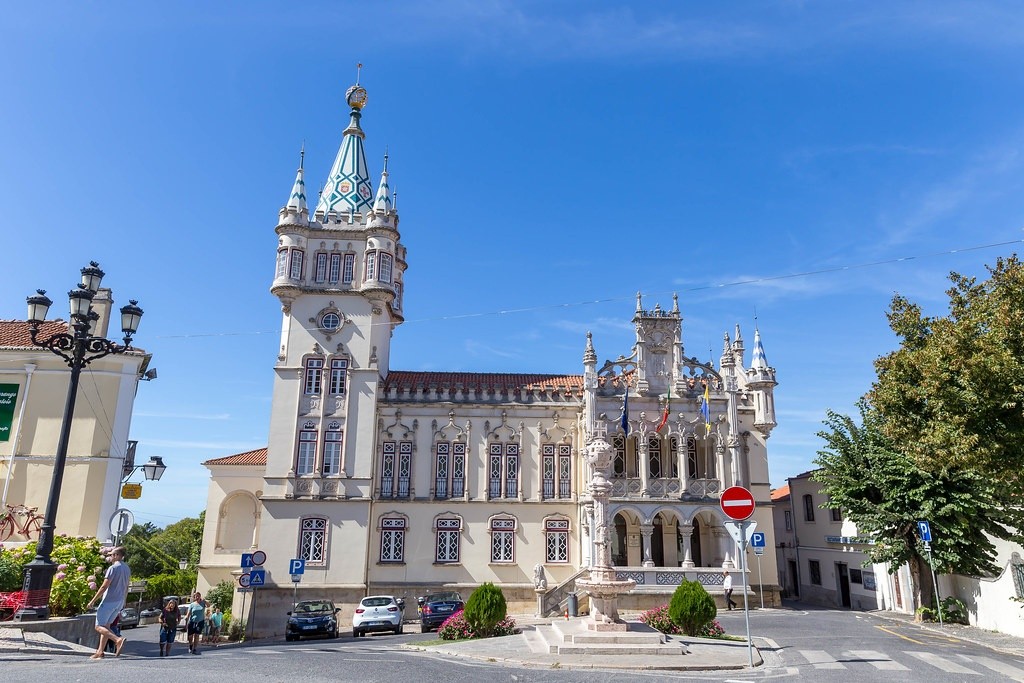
[567, 591, 578, 616]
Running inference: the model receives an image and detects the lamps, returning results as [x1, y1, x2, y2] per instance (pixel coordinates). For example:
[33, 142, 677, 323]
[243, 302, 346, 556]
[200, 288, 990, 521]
[139, 368, 157, 381]
[178, 558, 194, 569]
[120, 453, 166, 484]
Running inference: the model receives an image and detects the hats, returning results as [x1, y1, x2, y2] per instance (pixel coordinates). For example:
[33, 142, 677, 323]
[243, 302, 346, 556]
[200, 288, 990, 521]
[108, 549, 126, 555]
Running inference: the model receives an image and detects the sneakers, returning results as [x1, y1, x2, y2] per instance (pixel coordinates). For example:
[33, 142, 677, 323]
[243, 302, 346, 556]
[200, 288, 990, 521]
[90, 653, 104, 659]
[115, 637, 127, 658]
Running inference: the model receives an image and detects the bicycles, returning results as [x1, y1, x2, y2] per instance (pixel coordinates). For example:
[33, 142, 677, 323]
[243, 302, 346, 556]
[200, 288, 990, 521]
[0, 503, 45, 542]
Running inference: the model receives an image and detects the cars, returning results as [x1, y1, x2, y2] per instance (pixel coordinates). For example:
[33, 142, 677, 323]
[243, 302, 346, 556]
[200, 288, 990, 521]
[285, 599, 341, 642]
[353, 595, 405, 638]
[120, 607, 139, 629]
[421, 590, 465, 633]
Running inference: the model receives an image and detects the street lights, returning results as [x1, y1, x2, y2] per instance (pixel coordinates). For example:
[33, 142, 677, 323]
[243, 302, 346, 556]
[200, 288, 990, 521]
[13, 259, 144, 624]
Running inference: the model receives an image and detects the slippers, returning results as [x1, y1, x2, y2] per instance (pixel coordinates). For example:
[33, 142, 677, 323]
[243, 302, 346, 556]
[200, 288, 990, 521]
[194, 651, 197, 653]
[189, 649, 192, 654]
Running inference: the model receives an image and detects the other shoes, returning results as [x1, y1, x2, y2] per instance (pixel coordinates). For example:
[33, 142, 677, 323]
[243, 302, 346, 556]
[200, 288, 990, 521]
[160, 651, 163, 656]
[726, 607, 731, 610]
[734, 603, 736, 607]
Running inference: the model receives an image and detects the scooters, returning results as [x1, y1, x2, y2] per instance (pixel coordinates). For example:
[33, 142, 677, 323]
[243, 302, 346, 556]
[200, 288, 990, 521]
[395, 591, 407, 620]
[413, 588, 430, 619]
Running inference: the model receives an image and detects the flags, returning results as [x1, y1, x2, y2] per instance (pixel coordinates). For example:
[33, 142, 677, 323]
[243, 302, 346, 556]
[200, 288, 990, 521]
[700, 384, 711, 432]
[655, 383, 671, 433]
[621, 387, 629, 438]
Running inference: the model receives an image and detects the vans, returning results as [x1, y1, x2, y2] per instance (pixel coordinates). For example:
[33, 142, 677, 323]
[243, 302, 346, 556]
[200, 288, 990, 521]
[176, 604, 191, 628]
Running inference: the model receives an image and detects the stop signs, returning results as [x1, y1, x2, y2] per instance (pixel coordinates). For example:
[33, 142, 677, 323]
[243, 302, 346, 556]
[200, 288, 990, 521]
[721, 486, 756, 521]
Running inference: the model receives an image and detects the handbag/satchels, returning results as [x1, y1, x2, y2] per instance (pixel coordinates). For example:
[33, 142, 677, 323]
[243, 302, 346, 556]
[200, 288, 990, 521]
[118, 610, 127, 621]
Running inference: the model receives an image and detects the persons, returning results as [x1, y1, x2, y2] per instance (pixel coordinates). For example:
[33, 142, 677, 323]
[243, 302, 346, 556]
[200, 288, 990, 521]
[201, 608, 223, 645]
[159, 599, 181, 657]
[184, 592, 210, 655]
[87, 546, 131, 661]
[723, 570, 737, 611]
